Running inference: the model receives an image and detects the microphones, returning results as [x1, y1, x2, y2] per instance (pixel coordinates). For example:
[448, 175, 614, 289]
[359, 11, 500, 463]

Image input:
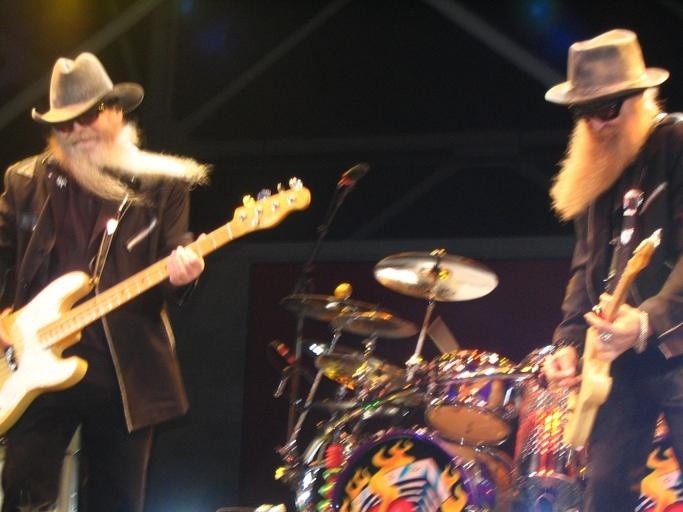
[335, 160, 373, 190]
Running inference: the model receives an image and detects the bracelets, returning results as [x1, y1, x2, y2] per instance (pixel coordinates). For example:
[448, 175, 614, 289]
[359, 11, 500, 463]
[632, 309, 649, 355]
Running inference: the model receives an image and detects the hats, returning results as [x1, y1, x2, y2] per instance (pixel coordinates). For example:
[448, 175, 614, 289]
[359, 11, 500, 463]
[31, 52, 143, 124]
[544, 29, 669, 105]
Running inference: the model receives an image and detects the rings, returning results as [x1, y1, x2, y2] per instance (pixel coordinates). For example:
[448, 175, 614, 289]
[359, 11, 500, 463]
[598, 331, 615, 344]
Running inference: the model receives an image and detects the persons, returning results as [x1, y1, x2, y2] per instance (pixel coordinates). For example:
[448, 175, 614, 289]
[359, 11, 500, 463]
[545, 30, 682, 512]
[0, 52, 205, 511]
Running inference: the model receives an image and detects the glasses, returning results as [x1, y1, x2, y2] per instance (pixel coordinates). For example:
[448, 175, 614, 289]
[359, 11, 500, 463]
[567, 90, 644, 120]
[52, 98, 118, 130]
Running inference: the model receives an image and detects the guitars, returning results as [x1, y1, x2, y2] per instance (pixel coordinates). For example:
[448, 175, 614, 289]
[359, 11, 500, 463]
[564, 225, 663, 453]
[2, 178, 312, 441]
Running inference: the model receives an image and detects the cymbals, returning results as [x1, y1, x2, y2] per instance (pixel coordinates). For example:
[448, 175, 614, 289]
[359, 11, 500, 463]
[280, 284, 376, 322]
[313, 340, 421, 397]
[334, 307, 421, 342]
[376, 252, 502, 305]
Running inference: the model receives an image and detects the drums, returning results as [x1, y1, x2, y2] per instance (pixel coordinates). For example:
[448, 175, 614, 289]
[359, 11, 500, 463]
[291, 431, 508, 512]
[423, 347, 521, 446]
[516, 447, 588, 510]
